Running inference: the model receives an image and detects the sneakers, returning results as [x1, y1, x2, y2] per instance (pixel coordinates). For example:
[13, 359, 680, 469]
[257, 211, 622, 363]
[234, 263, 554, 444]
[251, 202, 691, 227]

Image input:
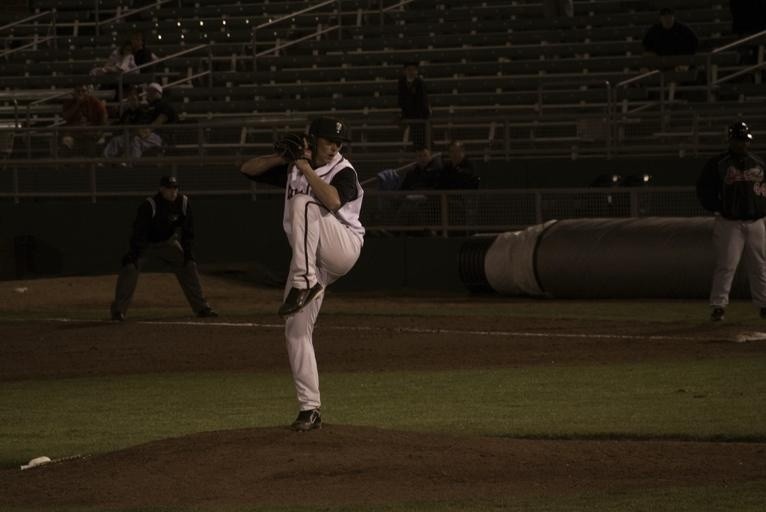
[197, 309, 219, 318]
[277, 279, 323, 317]
[290, 410, 323, 433]
[113, 313, 124, 321]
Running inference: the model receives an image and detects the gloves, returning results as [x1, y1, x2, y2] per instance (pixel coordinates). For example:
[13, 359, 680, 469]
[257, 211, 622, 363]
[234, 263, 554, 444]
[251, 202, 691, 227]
[122, 250, 142, 267]
[181, 245, 196, 265]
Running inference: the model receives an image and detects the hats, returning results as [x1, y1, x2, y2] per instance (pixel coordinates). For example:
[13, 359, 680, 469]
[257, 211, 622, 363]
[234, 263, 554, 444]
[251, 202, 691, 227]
[158, 175, 181, 189]
[310, 117, 352, 144]
[143, 83, 164, 94]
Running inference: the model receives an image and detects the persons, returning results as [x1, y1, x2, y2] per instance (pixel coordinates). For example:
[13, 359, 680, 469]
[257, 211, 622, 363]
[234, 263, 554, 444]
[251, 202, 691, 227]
[640, 8, 700, 101]
[55, 29, 175, 170]
[236, 116, 366, 433]
[108, 176, 221, 321]
[396, 58, 431, 152]
[435, 139, 480, 236]
[696, 118, 765, 321]
[397, 143, 438, 236]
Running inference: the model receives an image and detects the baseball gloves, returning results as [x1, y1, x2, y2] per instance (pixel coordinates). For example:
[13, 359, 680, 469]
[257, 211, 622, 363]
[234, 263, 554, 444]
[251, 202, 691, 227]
[275, 130, 307, 159]
